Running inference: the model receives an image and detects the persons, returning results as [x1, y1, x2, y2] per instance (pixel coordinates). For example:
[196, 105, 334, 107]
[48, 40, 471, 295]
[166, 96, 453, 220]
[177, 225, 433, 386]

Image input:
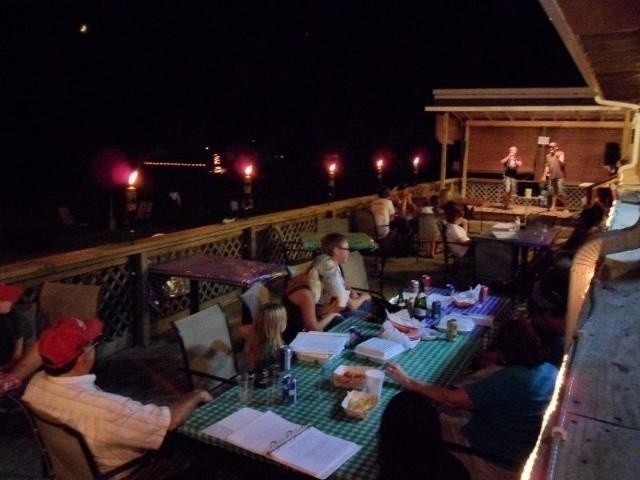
[446, 204, 474, 258]
[383, 319, 558, 479]
[306, 233, 372, 317]
[370, 182, 446, 259]
[526, 187, 612, 348]
[375, 389, 472, 479]
[280, 253, 345, 346]
[499, 145, 523, 209]
[22, 315, 212, 479]
[241, 301, 289, 374]
[603, 159, 625, 177]
[541, 142, 569, 214]
[0, 283, 45, 395]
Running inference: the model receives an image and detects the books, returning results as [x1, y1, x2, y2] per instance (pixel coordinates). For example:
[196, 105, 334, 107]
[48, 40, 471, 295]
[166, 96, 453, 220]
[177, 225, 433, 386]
[226, 408, 363, 480]
[289, 330, 351, 354]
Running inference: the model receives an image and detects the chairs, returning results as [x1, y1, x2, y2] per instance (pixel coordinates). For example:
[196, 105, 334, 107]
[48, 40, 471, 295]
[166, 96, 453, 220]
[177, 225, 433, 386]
[269, 190, 484, 259]
[471, 195, 609, 271]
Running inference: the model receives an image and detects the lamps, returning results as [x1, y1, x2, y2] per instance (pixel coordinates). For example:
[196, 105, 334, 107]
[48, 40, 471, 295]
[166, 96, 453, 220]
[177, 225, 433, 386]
[537, 136, 550, 149]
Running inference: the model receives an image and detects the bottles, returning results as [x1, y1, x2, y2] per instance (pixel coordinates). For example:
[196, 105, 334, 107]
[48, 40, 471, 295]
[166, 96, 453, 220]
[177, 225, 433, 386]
[408, 280, 419, 294]
[540, 225, 547, 240]
[416, 278, 424, 291]
[515, 217, 520, 231]
[413, 279, 427, 321]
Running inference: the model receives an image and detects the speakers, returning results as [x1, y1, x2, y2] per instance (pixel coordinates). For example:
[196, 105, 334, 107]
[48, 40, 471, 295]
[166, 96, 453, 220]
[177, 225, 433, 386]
[604, 142, 622, 166]
[450, 138, 466, 162]
[517, 181, 540, 197]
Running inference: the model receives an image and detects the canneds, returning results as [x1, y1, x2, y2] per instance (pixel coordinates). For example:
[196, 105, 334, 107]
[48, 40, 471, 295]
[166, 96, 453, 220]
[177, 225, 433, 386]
[282, 373, 297, 404]
[431, 300, 441, 320]
[420, 274, 433, 290]
[409, 279, 421, 293]
[446, 283, 457, 296]
[480, 286, 489, 301]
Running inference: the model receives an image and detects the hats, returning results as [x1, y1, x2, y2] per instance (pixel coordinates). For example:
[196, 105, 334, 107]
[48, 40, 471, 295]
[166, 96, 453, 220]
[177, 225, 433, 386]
[1, 282, 22, 301]
[38, 315, 103, 368]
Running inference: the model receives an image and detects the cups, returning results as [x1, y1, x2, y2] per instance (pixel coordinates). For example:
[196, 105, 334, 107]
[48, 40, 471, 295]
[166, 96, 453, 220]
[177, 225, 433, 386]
[480, 285, 489, 302]
[442, 281, 456, 296]
[237, 375, 255, 402]
[365, 370, 385, 400]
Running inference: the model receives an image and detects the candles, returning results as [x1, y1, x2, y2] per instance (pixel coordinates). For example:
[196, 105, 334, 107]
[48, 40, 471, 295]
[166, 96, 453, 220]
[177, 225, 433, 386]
[124, 169, 139, 210]
[374, 160, 384, 179]
[326, 165, 337, 184]
[242, 163, 255, 195]
[413, 156, 420, 177]
